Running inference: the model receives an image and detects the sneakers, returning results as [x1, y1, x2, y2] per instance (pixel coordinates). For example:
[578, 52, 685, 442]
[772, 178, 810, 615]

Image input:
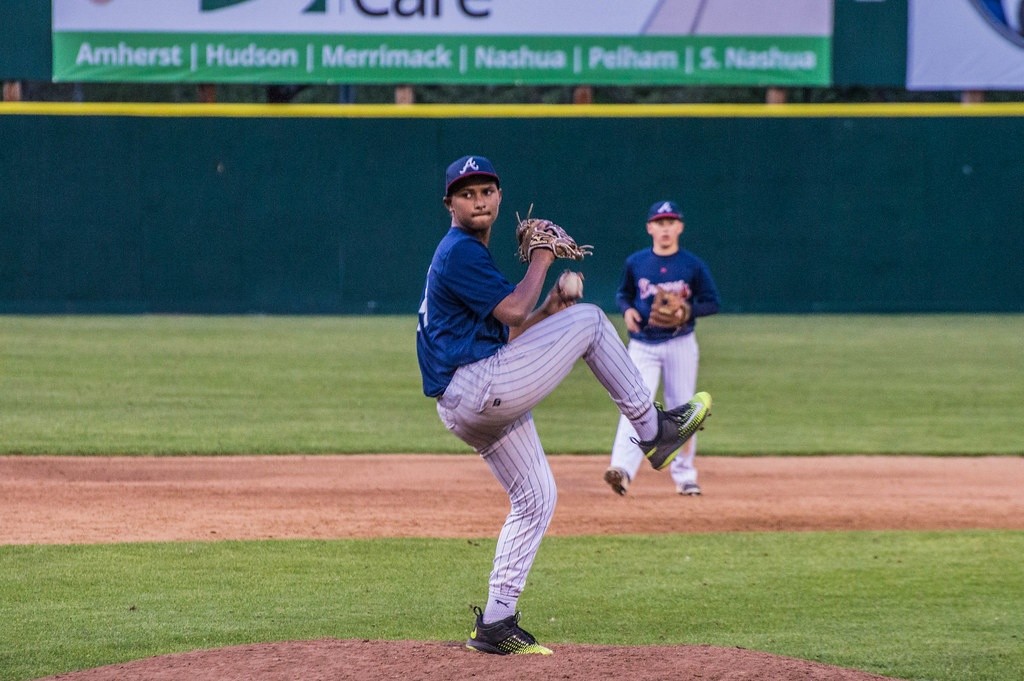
[604, 466, 629, 496]
[466, 605, 554, 656]
[630, 392, 712, 470]
[677, 482, 702, 495]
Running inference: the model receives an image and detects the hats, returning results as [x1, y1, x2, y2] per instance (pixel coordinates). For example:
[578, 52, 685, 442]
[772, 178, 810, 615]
[647, 199, 684, 222]
[445, 155, 500, 197]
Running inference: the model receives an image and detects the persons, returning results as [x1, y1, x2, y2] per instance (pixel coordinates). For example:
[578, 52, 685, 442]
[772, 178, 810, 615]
[413, 155, 714, 656]
[603, 200, 725, 498]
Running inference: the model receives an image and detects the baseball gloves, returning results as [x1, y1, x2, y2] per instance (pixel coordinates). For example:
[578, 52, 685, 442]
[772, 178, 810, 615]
[515, 217, 585, 262]
[647, 289, 692, 329]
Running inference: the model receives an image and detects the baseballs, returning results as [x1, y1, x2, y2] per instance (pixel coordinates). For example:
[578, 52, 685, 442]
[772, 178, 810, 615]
[556, 270, 585, 300]
